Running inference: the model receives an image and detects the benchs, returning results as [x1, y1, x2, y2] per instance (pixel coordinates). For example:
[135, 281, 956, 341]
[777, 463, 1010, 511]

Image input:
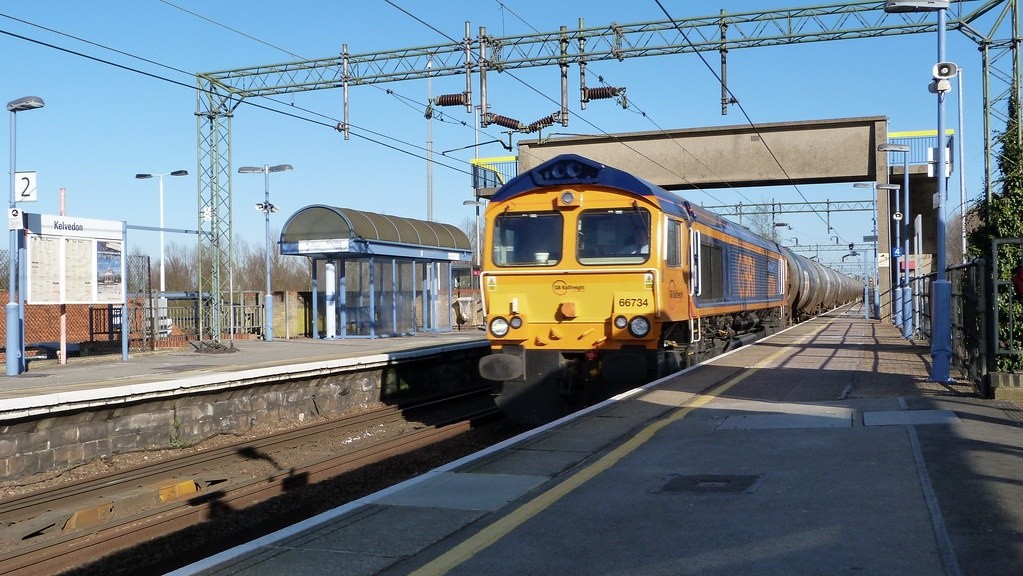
[337, 305, 393, 335]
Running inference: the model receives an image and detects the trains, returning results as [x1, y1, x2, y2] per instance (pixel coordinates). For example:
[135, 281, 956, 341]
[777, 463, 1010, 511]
[479, 152, 873, 409]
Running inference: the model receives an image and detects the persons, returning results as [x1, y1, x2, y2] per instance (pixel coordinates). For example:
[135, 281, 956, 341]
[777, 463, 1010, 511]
[614, 225, 648, 255]
[454, 275, 462, 290]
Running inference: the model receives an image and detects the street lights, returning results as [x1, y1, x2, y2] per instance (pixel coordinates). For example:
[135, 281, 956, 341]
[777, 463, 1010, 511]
[876, 184, 903, 327]
[136, 169, 189, 307]
[883, 0, 957, 384]
[853, 182, 881, 319]
[238, 164, 296, 341]
[878, 143, 913, 340]
[5, 95, 47, 376]
